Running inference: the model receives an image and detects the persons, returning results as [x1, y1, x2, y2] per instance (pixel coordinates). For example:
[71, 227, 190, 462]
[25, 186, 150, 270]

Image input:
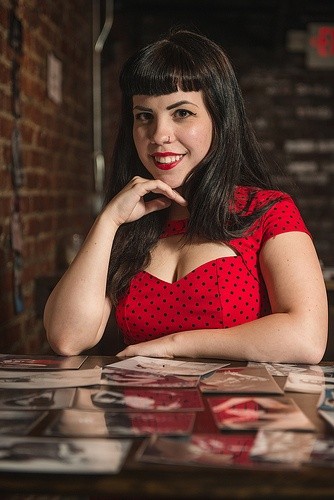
[44, 29, 329, 364]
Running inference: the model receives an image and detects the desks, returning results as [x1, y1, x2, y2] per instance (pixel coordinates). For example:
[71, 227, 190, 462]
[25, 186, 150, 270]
[0, 356, 334, 500]
